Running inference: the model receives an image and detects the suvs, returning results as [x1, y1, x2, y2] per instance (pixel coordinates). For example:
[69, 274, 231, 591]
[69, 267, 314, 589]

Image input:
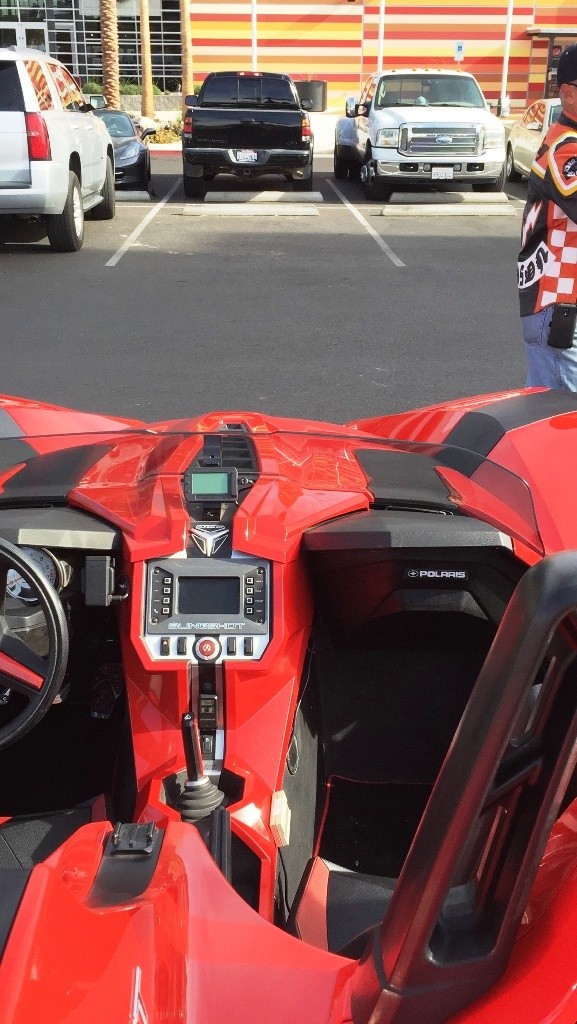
[0, 46, 115, 251]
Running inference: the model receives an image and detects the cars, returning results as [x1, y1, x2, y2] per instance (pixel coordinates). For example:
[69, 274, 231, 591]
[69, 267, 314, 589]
[505, 98, 563, 181]
[92, 109, 157, 191]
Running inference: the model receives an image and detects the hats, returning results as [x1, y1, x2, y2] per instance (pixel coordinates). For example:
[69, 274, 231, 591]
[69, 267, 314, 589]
[557, 44, 577, 88]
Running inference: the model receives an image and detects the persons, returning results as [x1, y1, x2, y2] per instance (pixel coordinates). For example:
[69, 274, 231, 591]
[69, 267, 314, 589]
[516, 42, 577, 400]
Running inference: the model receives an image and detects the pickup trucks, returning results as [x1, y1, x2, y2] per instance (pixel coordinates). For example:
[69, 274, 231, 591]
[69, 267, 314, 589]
[334, 68, 508, 200]
[181, 72, 315, 201]
